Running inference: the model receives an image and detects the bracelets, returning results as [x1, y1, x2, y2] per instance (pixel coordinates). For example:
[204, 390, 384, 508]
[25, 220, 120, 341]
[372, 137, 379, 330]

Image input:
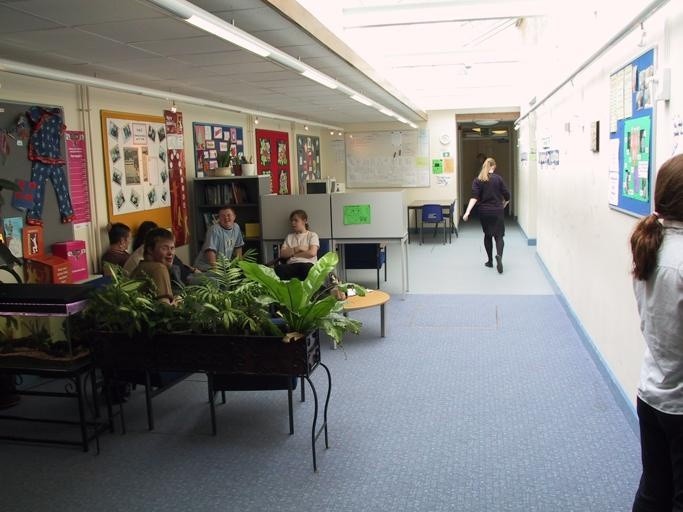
[192, 267, 197, 273]
[293, 247, 296, 254]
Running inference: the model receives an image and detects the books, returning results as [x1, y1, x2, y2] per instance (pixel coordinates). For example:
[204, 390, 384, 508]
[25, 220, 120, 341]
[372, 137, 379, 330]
[202, 182, 247, 230]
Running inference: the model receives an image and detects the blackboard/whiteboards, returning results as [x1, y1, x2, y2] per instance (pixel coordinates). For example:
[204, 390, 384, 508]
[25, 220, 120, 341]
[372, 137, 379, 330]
[343, 128, 431, 188]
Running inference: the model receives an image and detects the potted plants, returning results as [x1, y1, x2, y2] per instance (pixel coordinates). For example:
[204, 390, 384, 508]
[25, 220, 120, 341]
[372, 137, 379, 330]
[215, 138, 255, 177]
[82, 247, 366, 376]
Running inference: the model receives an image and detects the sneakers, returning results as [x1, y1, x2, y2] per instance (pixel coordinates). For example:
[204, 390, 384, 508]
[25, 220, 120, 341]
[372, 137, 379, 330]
[485, 262, 493, 267]
[495, 255, 503, 273]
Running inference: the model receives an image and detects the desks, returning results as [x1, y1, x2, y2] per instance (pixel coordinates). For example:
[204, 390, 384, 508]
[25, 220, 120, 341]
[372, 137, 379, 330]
[407, 200, 455, 244]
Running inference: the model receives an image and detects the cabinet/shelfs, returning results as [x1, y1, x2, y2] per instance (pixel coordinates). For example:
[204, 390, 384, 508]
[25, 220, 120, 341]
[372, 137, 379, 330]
[0, 282, 115, 452]
[191, 177, 270, 263]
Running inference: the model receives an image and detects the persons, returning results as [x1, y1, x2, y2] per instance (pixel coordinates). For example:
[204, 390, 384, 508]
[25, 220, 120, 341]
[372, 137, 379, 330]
[99, 222, 131, 275]
[628, 152, 683, 510]
[191, 205, 245, 273]
[134, 221, 230, 292]
[128, 228, 182, 307]
[274, 210, 319, 281]
[462, 157, 510, 274]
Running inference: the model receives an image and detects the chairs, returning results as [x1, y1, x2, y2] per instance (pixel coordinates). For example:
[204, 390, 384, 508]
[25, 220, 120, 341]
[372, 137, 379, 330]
[265, 238, 386, 289]
[421, 199, 456, 243]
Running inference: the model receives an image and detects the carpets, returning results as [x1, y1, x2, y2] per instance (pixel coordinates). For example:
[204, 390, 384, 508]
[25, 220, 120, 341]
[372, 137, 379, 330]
[0, 294, 643, 511]
